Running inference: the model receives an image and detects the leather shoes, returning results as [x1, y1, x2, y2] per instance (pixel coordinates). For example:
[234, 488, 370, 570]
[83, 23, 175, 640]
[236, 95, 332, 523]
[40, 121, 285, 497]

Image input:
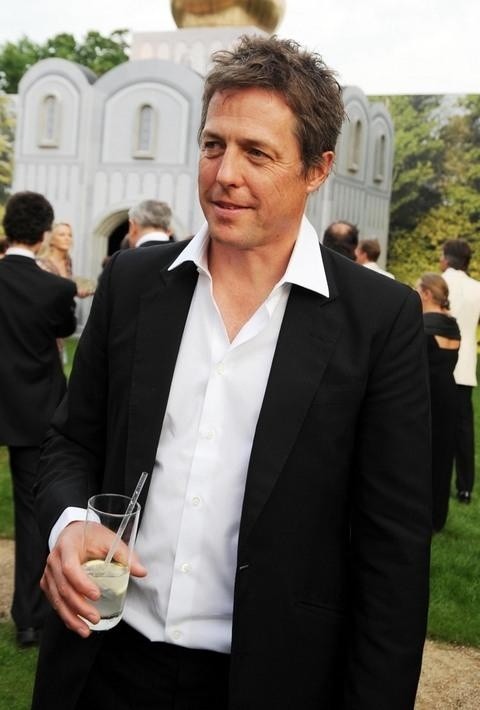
[17, 625, 39, 645]
[457, 490, 471, 502]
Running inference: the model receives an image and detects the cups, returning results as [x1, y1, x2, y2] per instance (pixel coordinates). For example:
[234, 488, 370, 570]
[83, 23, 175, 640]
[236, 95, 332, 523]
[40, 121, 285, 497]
[72, 493, 140, 632]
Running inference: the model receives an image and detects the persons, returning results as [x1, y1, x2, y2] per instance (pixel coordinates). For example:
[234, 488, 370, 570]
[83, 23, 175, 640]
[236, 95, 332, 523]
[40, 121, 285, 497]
[416, 273, 461, 528]
[0, 193, 78, 643]
[36, 223, 96, 369]
[29, 35, 435, 709]
[356, 239, 395, 279]
[323, 222, 359, 262]
[32, 34, 431, 710]
[126, 200, 173, 247]
[440, 240, 478, 504]
